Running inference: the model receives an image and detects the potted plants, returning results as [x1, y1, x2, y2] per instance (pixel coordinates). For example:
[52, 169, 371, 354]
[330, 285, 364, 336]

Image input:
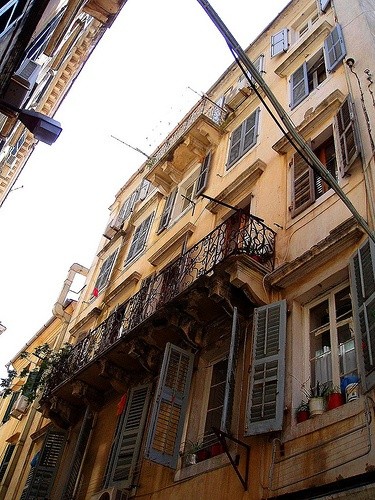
[291, 374, 329, 417]
[181, 439, 206, 467]
[325, 384, 343, 411]
[296, 403, 310, 423]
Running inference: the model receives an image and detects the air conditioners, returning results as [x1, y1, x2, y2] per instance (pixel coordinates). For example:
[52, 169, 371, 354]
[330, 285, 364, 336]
[224, 82, 250, 113]
[16, 52, 53, 87]
[88, 486, 130, 500]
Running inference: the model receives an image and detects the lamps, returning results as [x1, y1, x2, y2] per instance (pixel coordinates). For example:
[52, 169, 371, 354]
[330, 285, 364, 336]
[0, 98, 62, 147]
